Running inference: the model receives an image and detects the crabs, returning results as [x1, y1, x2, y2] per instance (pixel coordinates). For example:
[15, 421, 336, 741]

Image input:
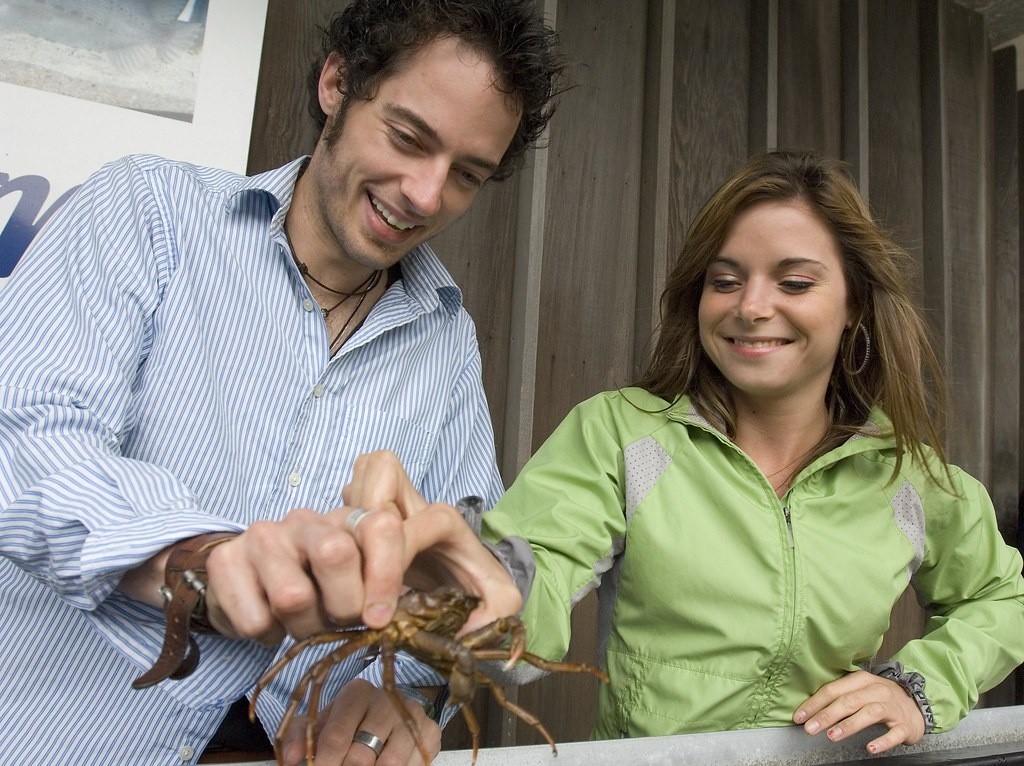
[249, 584, 609, 766]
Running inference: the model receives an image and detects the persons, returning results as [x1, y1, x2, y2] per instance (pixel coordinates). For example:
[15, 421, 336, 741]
[1, 1, 574, 766]
[341, 149, 1024, 754]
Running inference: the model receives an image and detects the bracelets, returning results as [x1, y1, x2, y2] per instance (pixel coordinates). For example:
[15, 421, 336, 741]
[389, 683, 434, 717]
[133, 532, 239, 692]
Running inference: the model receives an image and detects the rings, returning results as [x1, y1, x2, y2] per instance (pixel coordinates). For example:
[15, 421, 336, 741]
[354, 732, 383, 756]
[347, 510, 372, 532]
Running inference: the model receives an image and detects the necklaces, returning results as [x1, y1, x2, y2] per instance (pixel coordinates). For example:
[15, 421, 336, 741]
[283, 220, 383, 348]
